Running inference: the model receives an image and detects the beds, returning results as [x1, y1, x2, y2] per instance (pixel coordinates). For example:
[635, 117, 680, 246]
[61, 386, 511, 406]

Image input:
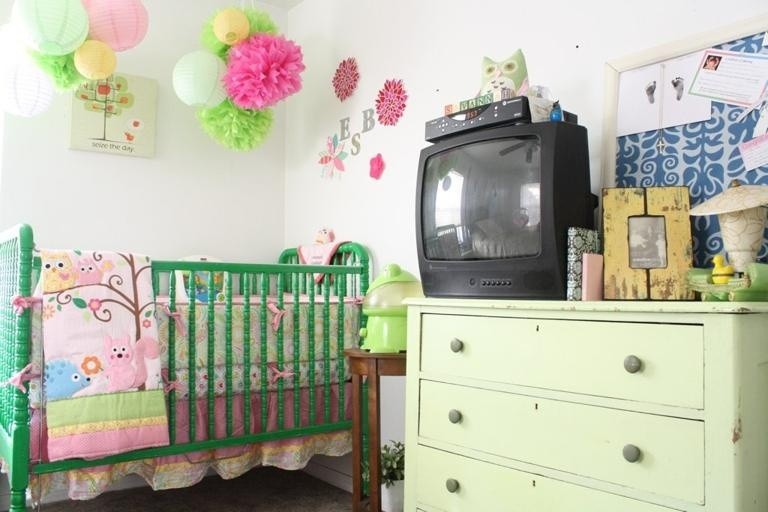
[0, 224, 373, 512]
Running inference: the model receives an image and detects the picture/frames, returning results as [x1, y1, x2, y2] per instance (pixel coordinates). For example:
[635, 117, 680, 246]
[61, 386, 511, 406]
[598, 184, 694, 302]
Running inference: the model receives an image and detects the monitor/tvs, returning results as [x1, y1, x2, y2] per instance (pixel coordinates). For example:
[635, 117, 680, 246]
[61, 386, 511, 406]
[416, 122, 598, 300]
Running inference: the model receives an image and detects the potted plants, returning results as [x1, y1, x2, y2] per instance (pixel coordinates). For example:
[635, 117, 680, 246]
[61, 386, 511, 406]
[358, 438, 405, 511]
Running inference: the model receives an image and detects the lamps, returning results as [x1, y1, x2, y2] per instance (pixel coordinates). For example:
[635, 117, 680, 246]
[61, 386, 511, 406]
[0, 0, 149, 121]
[170, 1, 252, 112]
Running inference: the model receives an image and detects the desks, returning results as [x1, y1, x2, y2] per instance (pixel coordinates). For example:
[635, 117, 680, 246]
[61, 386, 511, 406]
[341, 346, 406, 512]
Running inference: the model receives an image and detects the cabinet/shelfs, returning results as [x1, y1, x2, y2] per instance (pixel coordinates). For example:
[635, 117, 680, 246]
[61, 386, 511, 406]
[400, 297, 766, 512]
